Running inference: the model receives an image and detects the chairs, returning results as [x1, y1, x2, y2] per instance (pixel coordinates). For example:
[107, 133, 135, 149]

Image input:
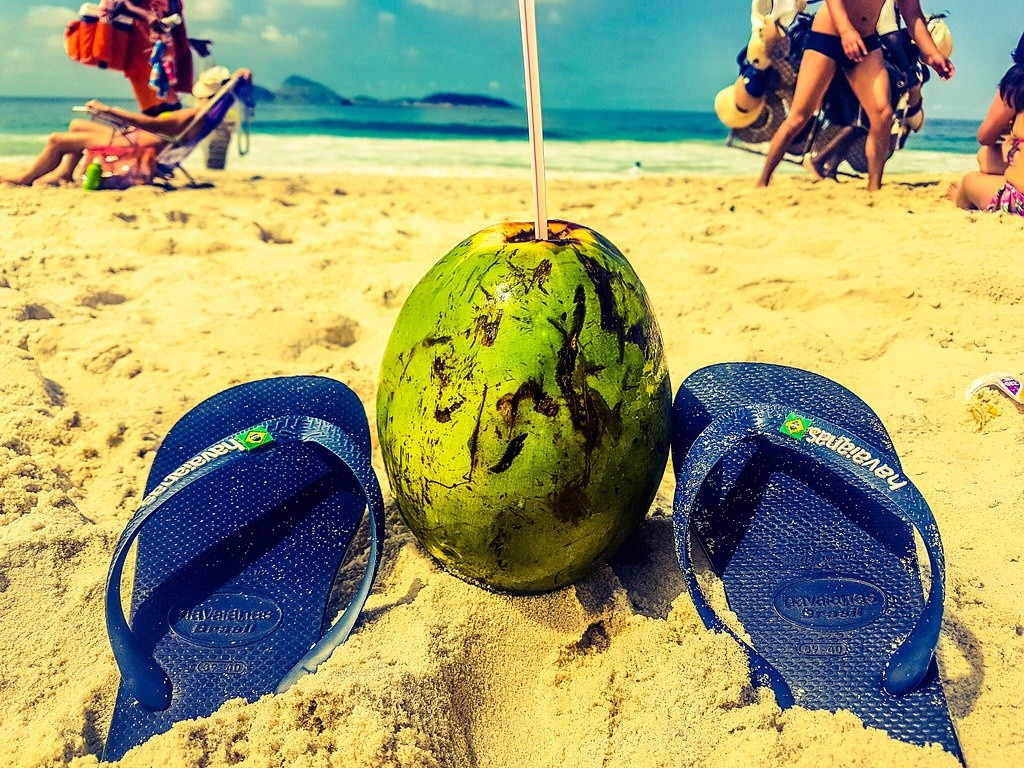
[72, 75, 254, 189]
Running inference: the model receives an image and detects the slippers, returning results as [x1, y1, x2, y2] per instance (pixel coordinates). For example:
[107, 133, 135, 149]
[669, 362, 966, 768]
[100, 377, 388, 762]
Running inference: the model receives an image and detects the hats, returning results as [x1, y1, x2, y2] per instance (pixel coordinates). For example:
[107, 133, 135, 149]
[715, 0, 954, 173]
[192, 66, 230, 98]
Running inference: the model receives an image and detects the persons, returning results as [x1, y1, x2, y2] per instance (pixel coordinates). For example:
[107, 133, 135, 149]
[944, 29, 1024, 219]
[62, 1, 215, 120]
[753, 1, 957, 194]
[0, 63, 234, 190]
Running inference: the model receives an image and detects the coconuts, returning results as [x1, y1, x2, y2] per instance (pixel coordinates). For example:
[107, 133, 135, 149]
[377, 219, 672, 596]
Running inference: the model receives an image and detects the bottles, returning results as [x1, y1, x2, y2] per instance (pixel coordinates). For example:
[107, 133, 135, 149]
[83, 157, 103, 190]
